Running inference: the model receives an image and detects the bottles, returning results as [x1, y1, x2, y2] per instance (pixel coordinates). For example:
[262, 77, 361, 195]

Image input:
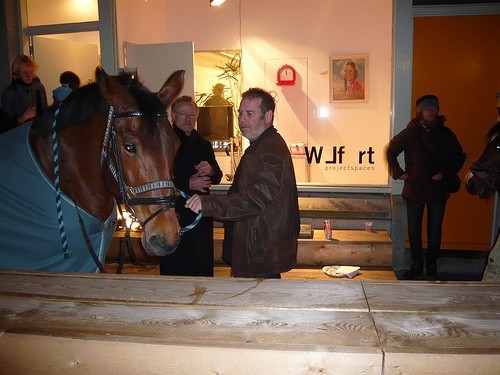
[209, 136, 230, 149]
[323, 219, 332, 240]
[198, 160, 210, 196]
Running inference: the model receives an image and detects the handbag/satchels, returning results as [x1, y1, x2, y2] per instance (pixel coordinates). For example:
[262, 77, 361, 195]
[448, 174, 461, 193]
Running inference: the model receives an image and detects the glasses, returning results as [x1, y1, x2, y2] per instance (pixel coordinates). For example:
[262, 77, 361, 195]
[173, 111, 197, 118]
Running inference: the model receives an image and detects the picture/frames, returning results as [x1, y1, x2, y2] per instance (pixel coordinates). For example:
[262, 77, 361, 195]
[329, 54, 369, 104]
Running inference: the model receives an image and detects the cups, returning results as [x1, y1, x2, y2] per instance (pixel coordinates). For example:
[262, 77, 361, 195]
[365, 222, 373, 232]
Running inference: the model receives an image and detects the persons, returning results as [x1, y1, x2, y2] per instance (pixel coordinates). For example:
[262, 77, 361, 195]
[159, 95, 223, 276]
[204, 83, 235, 138]
[53, 71, 80, 102]
[384, 94, 466, 281]
[462, 92, 500, 283]
[184, 88, 300, 279]
[0, 54, 49, 133]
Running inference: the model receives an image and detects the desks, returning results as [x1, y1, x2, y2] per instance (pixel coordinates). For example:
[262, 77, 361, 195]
[361, 279, 500, 375]
[0, 268, 384, 375]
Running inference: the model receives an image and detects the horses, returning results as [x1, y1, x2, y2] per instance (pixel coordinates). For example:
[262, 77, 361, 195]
[0, 66, 186, 275]
[465, 120, 500, 199]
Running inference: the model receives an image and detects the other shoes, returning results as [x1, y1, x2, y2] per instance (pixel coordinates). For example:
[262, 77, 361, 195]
[402, 263, 423, 280]
[426, 262, 437, 275]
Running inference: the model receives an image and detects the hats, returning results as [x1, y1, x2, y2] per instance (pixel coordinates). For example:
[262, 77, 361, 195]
[415, 95, 440, 114]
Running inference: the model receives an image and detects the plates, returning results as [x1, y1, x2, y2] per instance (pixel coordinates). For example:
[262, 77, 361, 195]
[323, 265, 360, 278]
